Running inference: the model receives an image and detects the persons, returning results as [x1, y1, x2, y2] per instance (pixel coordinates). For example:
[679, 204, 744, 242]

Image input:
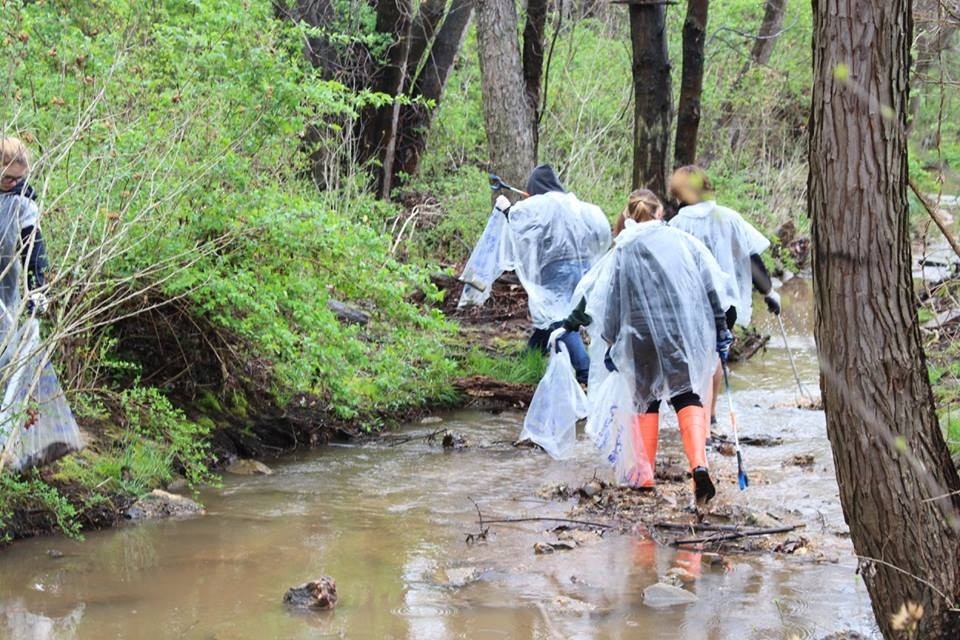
[495, 162, 782, 504]
[0, 136, 50, 472]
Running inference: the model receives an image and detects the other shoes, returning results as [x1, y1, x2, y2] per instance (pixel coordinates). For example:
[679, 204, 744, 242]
[624, 471, 657, 488]
[693, 465, 716, 503]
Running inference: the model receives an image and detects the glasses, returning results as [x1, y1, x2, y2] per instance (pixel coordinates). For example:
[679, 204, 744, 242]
[0, 175, 23, 185]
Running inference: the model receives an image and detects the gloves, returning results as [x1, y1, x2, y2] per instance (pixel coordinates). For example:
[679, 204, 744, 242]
[763, 288, 781, 315]
[603, 343, 619, 374]
[27, 292, 49, 315]
[546, 326, 570, 354]
[495, 194, 512, 213]
[716, 329, 733, 361]
